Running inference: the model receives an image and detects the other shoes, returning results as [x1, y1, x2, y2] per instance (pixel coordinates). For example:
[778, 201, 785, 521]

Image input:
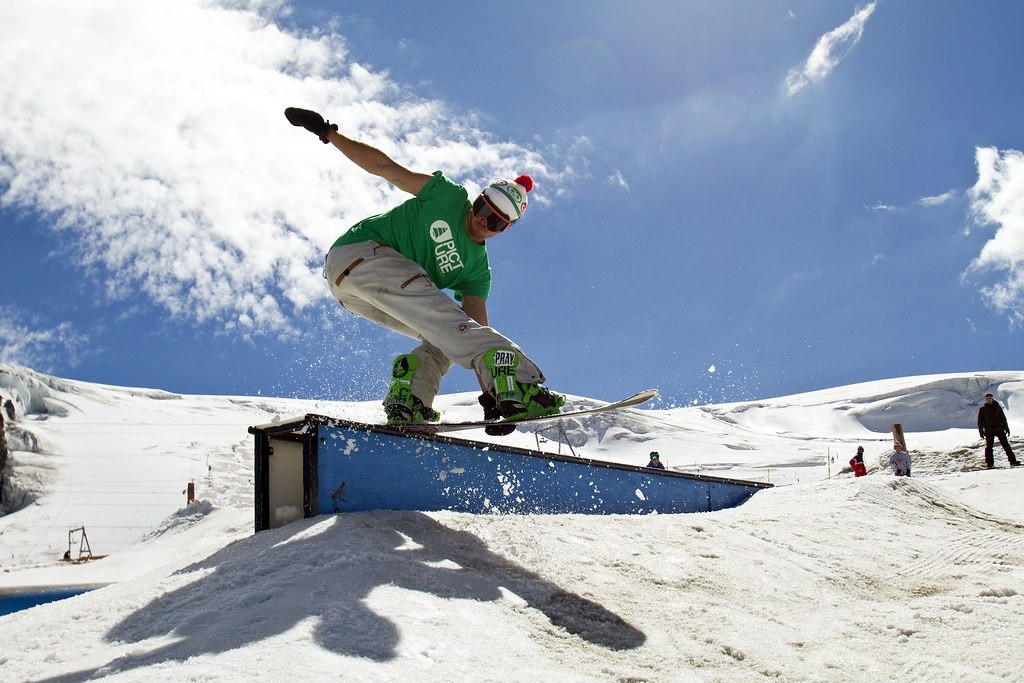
[986, 464, 996, 470]
[1013, 461, 1021, 465]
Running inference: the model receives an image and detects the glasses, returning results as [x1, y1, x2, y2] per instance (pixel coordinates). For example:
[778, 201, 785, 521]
[473, 194, 512, 233]
[651, 456, 659, 460]
[986, 398, 993, 400]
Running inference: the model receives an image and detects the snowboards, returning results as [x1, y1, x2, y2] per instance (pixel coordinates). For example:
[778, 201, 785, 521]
[369, 383, 663, 442]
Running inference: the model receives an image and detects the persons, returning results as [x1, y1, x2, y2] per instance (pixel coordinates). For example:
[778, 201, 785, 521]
[285, 106, 569, 435]
[977, 393, 1022, 467]
[889, 441, 911, 477]
[849, 446, 867, 476]
[647, 452, 665, 469]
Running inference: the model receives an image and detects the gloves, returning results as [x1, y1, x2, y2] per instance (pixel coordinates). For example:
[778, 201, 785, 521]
[1006, 429, 1010, 437]
[980, 431, 986, 439]
[284, 107, 339, 147]
[906, 468, 910, 476]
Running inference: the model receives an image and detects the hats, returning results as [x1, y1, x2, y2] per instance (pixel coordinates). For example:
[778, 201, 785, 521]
[893, 441, 903, 449]
[649, 451, 659, 456]
[985, 394, 993, 397]
[858, 446, 866, 452]
[481, 174, 533, 224]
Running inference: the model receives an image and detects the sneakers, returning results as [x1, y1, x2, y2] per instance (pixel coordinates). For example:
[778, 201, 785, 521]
[482, 348, 566, 421]
[382, 354, 441, 426]
